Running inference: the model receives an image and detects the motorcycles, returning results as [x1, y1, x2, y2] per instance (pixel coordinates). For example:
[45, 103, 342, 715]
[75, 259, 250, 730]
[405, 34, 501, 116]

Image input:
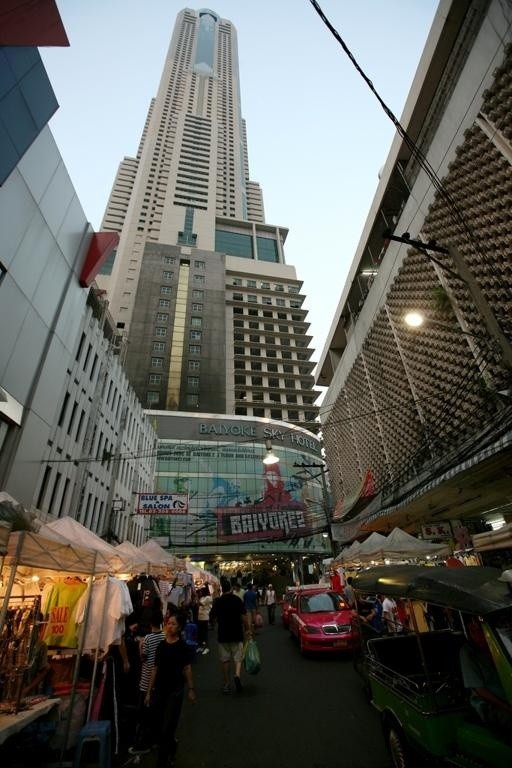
[351, 564, 512, 767]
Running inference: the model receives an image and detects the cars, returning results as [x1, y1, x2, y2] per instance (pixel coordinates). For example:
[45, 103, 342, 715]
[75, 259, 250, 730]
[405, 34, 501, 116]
[279, 586, 364, 657]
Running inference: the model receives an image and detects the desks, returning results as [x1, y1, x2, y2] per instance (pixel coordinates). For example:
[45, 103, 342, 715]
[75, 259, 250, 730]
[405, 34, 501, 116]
[1, 697, 62, 767]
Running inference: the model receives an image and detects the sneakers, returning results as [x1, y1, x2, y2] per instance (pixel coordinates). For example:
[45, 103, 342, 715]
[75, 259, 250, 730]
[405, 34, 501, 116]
[234, 675, 243, 694]
[223, 684, 231, 692]
[196, 648, 203, 652]
[128, 746, 150, 755]
[202, 648, 210, 654]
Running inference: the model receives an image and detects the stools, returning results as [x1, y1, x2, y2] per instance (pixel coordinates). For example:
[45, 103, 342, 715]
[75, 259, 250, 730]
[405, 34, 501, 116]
[74, 719, 113, 768]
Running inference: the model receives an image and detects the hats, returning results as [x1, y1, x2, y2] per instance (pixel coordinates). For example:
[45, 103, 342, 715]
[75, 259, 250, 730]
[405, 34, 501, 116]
[269, 584, 272, 587]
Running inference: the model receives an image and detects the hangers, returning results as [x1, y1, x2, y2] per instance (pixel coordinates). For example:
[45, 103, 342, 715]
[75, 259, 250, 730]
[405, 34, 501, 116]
[64, 576, 81, 584]
[7, 600, 34, 611]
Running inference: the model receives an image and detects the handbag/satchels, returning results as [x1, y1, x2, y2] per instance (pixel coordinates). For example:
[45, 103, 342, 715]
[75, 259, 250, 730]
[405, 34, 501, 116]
[135, 707, 155, 750]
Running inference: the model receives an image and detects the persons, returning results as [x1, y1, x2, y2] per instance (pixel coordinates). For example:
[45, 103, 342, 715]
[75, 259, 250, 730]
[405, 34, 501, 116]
[381, 594, 403, 636]
[352, 600, 377, 624]
[343, 577, 356, 606]
[258, 465, 294, 507]
[89, 581, 278, 768]
[458, 621, 512, 746]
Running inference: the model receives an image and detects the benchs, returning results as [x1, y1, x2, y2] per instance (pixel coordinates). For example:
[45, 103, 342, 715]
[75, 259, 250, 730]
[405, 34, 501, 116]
[367, 628, 456, 684]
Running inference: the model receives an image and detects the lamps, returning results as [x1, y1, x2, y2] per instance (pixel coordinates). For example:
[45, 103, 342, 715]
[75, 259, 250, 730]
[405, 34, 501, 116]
[263, 439, 278, 466]
